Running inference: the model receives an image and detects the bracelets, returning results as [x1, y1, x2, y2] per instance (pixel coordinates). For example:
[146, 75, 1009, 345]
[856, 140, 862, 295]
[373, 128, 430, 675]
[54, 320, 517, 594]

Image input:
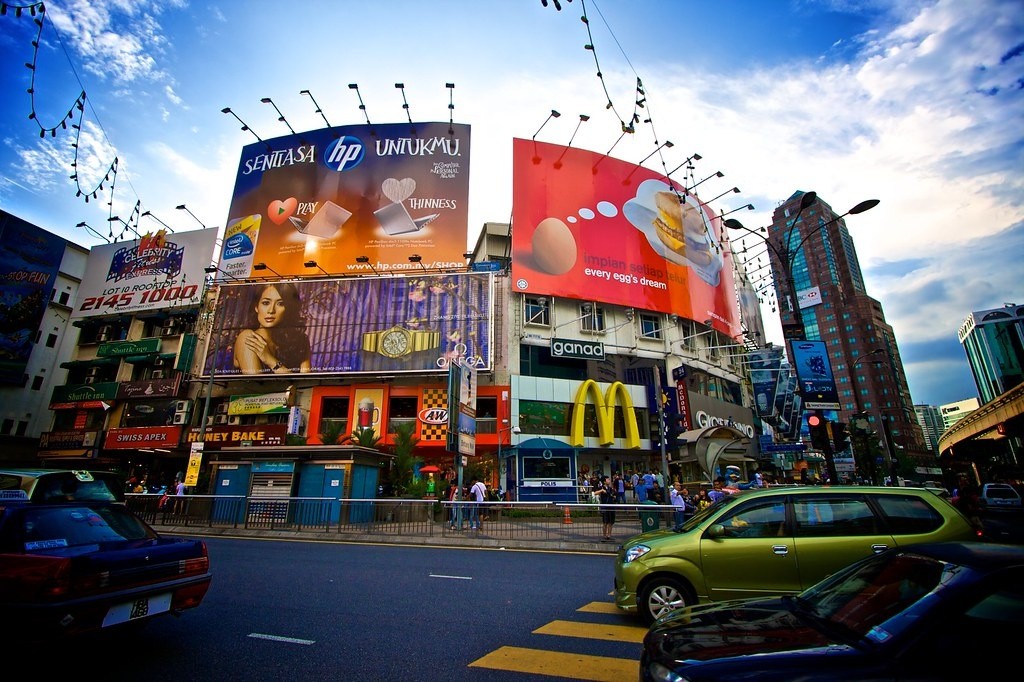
[270, 361, 283, 373]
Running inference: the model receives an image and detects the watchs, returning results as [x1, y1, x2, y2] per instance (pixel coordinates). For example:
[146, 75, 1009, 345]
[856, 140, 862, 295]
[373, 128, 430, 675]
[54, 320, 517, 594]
[362, 323, 442, 359]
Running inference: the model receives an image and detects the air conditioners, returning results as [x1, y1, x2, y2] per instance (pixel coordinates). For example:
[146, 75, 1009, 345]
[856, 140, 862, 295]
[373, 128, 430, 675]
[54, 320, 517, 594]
[85, 377, 94, 384]
[86, 367, 96, 376]
[154, 359, 163, 365]
[160, 318, 174, 336]
[173, 401, 242, 426]
[95, 325, 112, 342]
[152, 370, 163, 379]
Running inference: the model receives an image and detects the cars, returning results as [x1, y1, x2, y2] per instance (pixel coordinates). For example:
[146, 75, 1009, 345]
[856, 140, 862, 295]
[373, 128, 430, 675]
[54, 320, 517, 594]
[0, 490, 213, 631]
[639, 542, 1024, 682]
[924, 487, 952, 504]
[614, 486, 987, 628]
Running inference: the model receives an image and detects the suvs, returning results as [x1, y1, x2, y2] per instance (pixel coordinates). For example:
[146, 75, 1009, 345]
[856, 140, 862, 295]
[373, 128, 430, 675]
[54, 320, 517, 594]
[976, 482, 1022, 512]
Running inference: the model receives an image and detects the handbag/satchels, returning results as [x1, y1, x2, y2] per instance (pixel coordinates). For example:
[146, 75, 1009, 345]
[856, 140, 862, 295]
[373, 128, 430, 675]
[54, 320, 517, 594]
[483, 498, 489, 508]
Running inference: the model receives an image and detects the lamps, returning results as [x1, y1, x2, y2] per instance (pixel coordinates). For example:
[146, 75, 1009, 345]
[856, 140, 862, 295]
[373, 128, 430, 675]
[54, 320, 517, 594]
[204, 265, 238, 280]
[142, 210, 173, 233]
[299, 89, 338, 139]
[221, 107, 272, 152]
[534, 110, 783, 292]
[253, 263, 282, 279]
[348, 84, 375, 135]
[356, 255, 378, 274]
[394, 82, 416, 134]
[261, 98, 306, 146]
[446, 82, 455, 134]
[76, 222, 110, 242]
[409, 254, 426, 273]
[108, 216, 141, 238]
[175, 205, 205, 227]
[304, 261, 328, 275]
[520, 296, 792, 373]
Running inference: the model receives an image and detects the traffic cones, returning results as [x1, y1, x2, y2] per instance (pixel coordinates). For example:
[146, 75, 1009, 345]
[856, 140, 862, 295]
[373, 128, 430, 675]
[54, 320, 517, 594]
[563, 505, 573, 524]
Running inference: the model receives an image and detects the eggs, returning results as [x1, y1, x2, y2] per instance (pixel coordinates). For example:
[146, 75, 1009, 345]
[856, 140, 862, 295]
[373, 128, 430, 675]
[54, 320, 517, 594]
[532, 217, 577, 275]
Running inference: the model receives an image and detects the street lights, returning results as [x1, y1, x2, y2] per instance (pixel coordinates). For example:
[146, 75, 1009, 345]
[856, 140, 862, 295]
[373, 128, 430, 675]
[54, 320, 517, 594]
[724, 192, 879, 485]
[845, 349, 884, 485]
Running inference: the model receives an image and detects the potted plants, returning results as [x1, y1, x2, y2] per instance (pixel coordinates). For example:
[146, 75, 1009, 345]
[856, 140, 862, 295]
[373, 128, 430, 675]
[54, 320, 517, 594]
[375, 478, 450, 522]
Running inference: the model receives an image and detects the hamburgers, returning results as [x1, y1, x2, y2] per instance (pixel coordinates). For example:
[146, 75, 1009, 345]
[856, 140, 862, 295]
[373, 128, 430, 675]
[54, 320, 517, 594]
[652, 191, 714, 266]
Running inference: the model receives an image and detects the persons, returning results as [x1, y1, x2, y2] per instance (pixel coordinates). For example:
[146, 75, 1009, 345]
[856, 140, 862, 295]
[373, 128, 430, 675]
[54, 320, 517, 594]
[898, 477, 905, 487]
[754, 468, 875, 537]
[439, 474, 490, 530]
[126, 465, 189, 515]
[884, 478, 893, 487]
[593, 475, 616, 542]
[578, 471, 731, 527]
[720, 512, 762, 538]
[233, 284, 313, 375]
[957, 476, 974, 510]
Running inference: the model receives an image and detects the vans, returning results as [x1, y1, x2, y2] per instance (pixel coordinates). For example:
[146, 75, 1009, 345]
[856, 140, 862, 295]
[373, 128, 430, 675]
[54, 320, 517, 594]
[0, 468, 129, 506]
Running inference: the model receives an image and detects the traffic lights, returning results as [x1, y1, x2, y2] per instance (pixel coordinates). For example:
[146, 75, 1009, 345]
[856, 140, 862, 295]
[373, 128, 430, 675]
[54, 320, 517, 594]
[806, 414, 825, 448]
[667, 411, 687, 450]
[830, 420, 851, 452]
[783, 323, 805, 338]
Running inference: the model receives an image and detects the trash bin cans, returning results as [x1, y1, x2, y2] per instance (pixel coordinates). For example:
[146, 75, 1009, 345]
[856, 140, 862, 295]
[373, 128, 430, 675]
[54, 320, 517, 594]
[640, 499, 659, 533]
